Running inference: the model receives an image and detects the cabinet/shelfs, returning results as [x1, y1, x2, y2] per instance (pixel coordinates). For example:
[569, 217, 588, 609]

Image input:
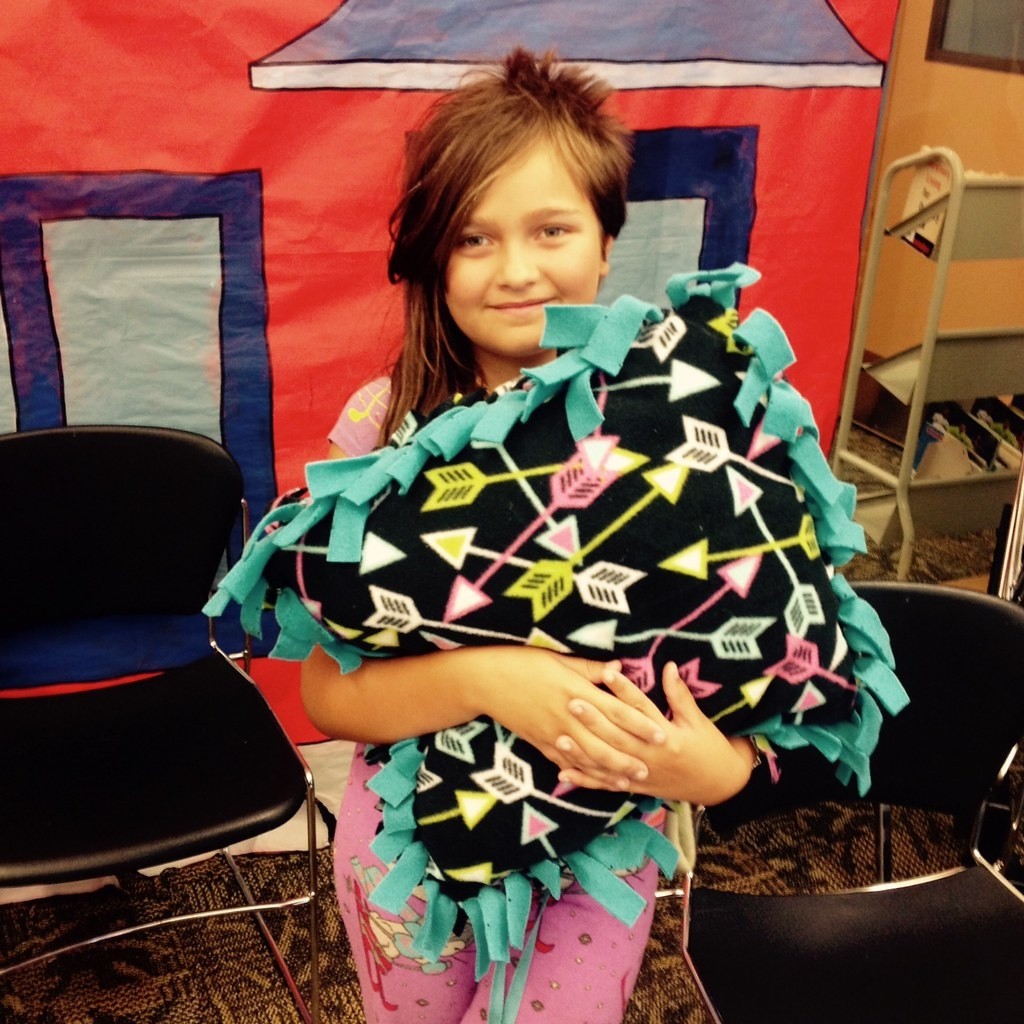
[827, 138, 1024, 584]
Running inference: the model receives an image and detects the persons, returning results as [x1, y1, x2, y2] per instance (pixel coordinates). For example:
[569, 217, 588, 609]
[302, 47, 767, 1024]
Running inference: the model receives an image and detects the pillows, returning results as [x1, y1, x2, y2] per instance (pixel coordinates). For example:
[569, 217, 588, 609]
[207, 261, 912, 979]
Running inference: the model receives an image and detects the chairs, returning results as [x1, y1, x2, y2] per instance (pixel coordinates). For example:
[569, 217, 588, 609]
[0, 422, 325, 1024]
[681, 580, 1024, 1022]
